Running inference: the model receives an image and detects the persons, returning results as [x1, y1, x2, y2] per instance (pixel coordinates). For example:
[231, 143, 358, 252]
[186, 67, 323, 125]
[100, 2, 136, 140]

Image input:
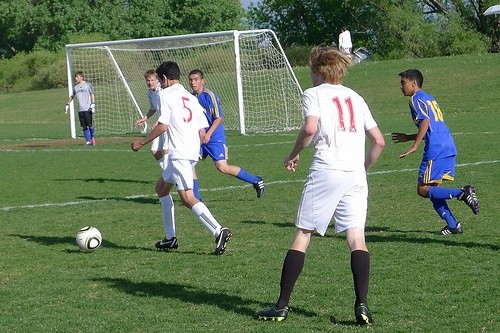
[136, 68, 169, 171]
[391, 71, 480, 236]
[64, 71, 95, 146]
[131, 60, 233, 255]
[189, 69, 264, 203]
[256, 47, 386, 327]
[338, 27, 352, 54]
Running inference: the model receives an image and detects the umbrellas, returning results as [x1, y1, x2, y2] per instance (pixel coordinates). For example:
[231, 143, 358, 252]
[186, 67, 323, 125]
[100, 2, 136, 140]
[483, 3, 500, 17]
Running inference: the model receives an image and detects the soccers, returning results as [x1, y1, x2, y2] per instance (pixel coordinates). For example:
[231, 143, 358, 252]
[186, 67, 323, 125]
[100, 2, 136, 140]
[76, 226, 102, 251]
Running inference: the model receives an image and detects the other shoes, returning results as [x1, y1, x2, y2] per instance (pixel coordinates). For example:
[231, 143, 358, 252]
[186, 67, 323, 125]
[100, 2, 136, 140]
[84, 141, 91, 147]
[92, 138, 96, 145]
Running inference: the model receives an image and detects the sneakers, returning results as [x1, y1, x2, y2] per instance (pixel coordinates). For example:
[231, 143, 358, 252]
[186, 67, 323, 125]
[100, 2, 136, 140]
[257, 305, 290, 321]
[354, 303, 375, 325]
[155, 237, 179, 249]
[254, 176, 265, 198]
[458, 185, 479, 215]
[438, 221, 463, 235]
[215, 227, 233, 255]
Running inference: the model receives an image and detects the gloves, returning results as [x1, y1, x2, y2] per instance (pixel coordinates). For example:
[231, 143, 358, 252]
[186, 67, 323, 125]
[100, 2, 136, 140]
[64, 104, 69, 114]
[89, 102, 96, 113]
